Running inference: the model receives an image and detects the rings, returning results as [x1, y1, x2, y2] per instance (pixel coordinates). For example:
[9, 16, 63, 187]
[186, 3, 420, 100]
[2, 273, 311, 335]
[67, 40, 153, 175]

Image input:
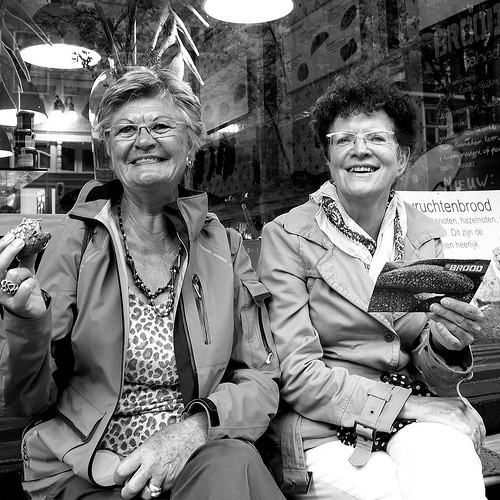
[1, 278, 19, 297]
[145, 483, 162, 497]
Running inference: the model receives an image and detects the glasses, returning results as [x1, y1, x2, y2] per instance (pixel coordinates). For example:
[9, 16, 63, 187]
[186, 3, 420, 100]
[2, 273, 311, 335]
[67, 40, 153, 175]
[326, 130, 396, 150]
[103, 122, 192, 142]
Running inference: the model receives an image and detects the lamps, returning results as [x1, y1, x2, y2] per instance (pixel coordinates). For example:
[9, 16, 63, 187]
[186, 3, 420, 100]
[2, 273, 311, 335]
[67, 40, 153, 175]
[202, 0, 295, 24]
[21, 31, 102, 69]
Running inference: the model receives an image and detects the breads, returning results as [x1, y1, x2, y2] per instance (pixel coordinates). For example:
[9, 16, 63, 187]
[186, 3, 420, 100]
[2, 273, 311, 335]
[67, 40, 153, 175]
[9, 217, 52, 261]
[376, 265, 474, 294]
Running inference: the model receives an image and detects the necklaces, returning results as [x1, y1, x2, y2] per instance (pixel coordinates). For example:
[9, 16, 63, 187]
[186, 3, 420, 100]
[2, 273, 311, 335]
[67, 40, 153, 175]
[116, 196, 187, 317]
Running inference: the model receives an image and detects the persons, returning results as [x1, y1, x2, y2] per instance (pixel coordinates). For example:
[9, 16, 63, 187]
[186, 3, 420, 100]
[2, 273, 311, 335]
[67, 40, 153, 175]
[0, 64, 287, 500]
[54, 95, 64, 111]
[256, 74, 489, 500]
